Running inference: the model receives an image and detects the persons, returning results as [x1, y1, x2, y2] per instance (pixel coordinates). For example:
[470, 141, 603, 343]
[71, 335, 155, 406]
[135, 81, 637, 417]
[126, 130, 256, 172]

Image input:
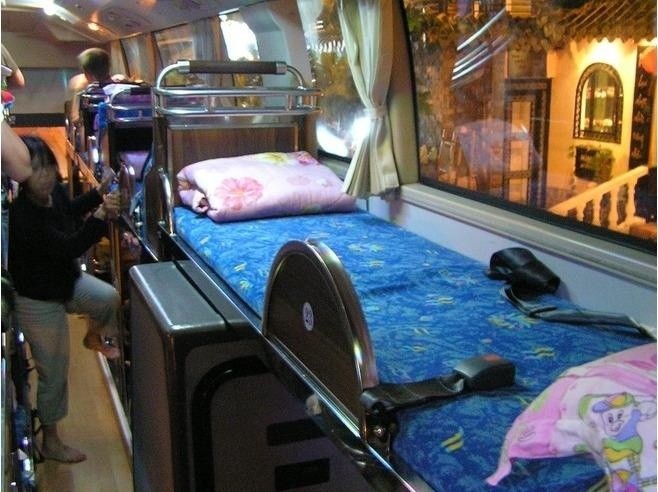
[1, 42, 25, 88]
[9, 136, 121, 463]
[73, 48, 124, 122]
[2, 114, 34, 182]
